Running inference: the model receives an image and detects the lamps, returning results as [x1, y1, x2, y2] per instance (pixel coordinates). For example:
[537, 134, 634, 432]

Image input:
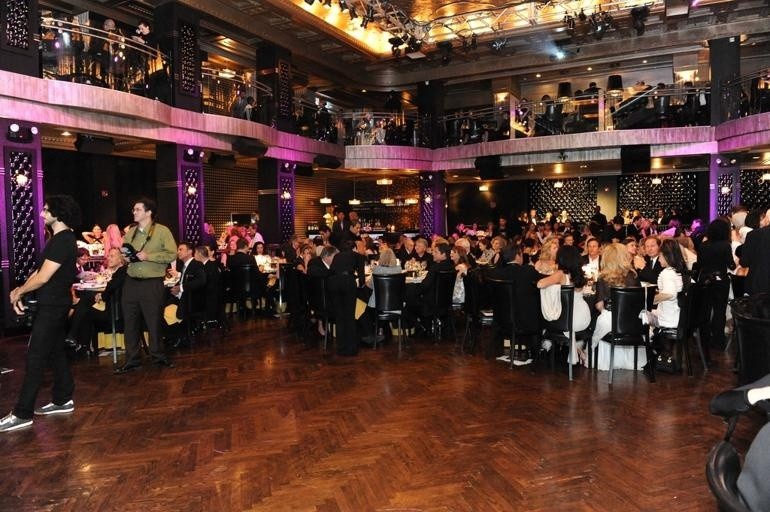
[302, 0, 375, 32]
[389, 35, 402, 57]
[438, 41, 452, 63]
[462, 36, 478, 51]
[563, 9, 654, 39]
[405, 36, 422, 54]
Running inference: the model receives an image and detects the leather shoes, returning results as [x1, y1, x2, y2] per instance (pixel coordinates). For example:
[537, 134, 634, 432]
[710, 389, 753, 416]
[112, 361, 143, 373]
[706, 441, 752, 511]
[369, 334, 386, 348]
[152, 357, 175, 369]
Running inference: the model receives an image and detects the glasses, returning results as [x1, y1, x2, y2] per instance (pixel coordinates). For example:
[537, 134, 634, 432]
[42, 208, 49, 214]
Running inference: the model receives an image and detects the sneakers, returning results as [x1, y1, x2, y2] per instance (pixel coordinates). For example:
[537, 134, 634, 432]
[33, 399, 75, 415]
[0, 410, 33, 432]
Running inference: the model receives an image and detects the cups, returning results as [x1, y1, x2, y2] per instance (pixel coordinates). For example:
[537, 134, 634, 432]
[77, 270, 112, 287]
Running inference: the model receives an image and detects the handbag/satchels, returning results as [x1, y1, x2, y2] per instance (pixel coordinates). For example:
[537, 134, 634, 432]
[638, 287, 658, 326]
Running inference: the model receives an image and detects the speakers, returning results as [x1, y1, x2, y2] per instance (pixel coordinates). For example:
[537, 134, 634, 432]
[235, 137, 267, 159]
[77, 134, 118, 158]
[621, 144, 651, 174]
[476, 155, 497, 181]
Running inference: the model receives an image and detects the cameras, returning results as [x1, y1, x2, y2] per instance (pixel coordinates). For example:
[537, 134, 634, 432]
[119, 242, 141, 263]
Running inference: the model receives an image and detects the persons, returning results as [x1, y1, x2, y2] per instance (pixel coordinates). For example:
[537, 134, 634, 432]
[517, 82, 600, 135]
[64, 224, 136, 354]
[297, 96, 509, 146]
[738, 421, 770, 511]
[164, 223, 276, 349]
[612, 80, 710, 128]
[281, 205, 770, 373]
[91, 18, 158, 78]
[0, 193, 81, 432]
[113, 197, 177, 375]
[230, 90, 257, 121]
[750, 70, 768, 113]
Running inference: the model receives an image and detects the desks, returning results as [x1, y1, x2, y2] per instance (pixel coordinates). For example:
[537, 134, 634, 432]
[220, 253, 289, 315]
[348, 261, 441, 347]
[567, 278, 674, 372]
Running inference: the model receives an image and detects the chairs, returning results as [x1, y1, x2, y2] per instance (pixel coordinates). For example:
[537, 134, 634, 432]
[41, 25, 770, 153]
[706, 373, 769, 506]
[209, 250, 280, 322]
[280, 252, 460, 357]
[87, 250, 208, 372]
[461, 253, 770, 383]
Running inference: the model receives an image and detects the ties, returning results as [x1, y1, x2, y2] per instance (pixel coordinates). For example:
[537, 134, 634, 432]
[181, 265, 187, 284]
